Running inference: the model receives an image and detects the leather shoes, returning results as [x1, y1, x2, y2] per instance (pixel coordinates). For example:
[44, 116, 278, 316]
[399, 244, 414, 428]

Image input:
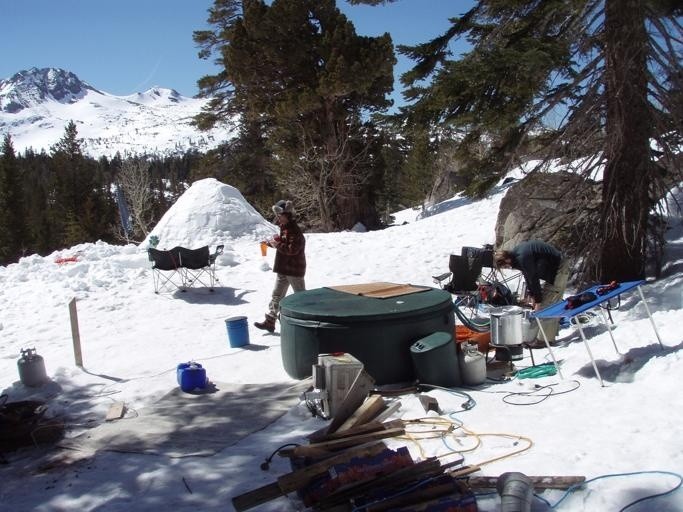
[526, 339, 553, 349]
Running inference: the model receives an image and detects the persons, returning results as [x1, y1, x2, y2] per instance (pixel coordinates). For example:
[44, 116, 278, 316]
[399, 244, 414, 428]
[252, 200, 307, 334]
[490, 242, 568, 350]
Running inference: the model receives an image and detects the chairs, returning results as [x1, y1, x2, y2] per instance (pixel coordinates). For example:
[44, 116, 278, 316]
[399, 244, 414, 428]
[433, 244, 496, 327]
[146, 243, 227, 294]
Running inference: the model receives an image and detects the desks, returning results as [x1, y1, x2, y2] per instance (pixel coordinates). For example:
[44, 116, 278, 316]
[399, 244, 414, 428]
[530, 279, 664, 389]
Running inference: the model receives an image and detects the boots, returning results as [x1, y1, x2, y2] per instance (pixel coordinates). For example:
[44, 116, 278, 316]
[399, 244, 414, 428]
[254, 317, 275, 334]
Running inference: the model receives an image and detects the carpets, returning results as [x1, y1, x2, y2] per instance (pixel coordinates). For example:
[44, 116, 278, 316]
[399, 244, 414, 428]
[55, 376, 316, 460]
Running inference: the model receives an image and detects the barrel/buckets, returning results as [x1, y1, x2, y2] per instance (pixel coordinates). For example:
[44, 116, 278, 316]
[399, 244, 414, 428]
[178, 361, 211, 391]
[410, 331, 461, 387]
[224, 316, 250, 349]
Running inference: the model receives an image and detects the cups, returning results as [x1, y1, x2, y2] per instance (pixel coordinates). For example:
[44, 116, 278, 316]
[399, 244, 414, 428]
[260, 241, 268, 256]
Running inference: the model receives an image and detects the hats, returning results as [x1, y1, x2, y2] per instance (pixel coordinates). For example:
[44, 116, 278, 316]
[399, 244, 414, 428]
[271, 198, 296, 215]
